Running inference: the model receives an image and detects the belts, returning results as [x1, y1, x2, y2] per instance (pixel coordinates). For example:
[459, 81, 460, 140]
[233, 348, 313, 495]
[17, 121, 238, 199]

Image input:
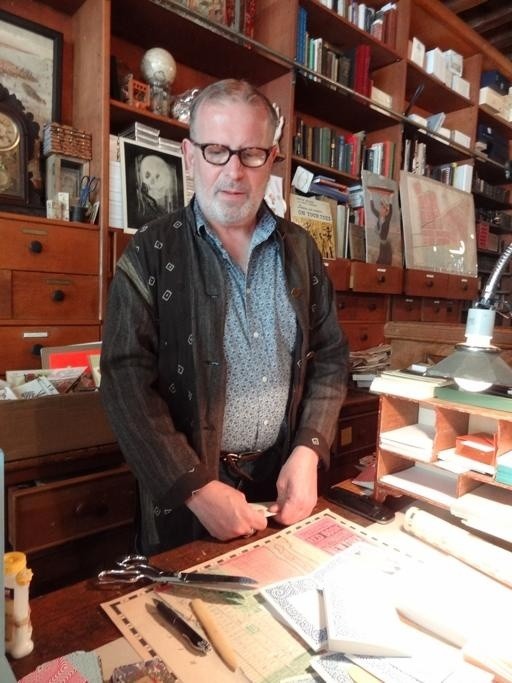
[219, 450, 268, 481]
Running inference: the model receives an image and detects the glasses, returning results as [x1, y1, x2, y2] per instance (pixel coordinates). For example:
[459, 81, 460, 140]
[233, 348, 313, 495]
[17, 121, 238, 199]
[188, 133, 275, 168]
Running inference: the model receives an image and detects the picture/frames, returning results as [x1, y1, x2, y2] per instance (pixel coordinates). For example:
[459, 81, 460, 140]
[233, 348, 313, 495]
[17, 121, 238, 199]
[0, 7, 62, 209]
[398, 174, 479, 279]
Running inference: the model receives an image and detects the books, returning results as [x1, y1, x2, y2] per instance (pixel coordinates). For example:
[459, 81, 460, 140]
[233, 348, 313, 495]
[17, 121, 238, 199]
[352, 367, 512, 546]
[479, 70, 512, 165]
[323, 0, 383, 40]
[294, 8, 373, 106]
[293, 118, 394, 181]
[289, 176, 366, 261]
[402, 138, 474, 193]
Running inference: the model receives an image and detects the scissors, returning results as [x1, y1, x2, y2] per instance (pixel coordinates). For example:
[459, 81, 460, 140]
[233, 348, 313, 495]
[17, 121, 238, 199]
[80, 176, 98, 208]
[98, 554, 258, 585]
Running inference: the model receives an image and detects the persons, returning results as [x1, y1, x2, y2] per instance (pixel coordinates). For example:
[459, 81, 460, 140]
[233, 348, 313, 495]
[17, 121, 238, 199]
[99, 72, 354, 559]
[366, 185, 399, 265]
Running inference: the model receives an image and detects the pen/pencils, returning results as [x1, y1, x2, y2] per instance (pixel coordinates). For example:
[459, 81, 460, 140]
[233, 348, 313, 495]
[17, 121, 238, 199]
[191, 599, 238, 669]
[396, 607, 465, 648]
[151, 598, 211, 654]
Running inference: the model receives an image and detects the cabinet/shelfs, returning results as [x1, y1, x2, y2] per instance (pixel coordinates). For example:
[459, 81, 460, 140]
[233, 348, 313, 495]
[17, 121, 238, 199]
[0, 211, 137, 597]
[73, 2, 292, 226]
[403, 1, 511, 268]
[323, 261, 482, 360]
[289, 3, 402, 265]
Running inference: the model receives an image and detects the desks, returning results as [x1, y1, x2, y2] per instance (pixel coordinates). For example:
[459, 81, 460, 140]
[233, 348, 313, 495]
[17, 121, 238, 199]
[3, 492, 510, 682]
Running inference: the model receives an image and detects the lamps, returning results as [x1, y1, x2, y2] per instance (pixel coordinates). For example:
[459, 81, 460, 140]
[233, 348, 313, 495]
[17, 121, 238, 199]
[424, 237, 512, 393]
